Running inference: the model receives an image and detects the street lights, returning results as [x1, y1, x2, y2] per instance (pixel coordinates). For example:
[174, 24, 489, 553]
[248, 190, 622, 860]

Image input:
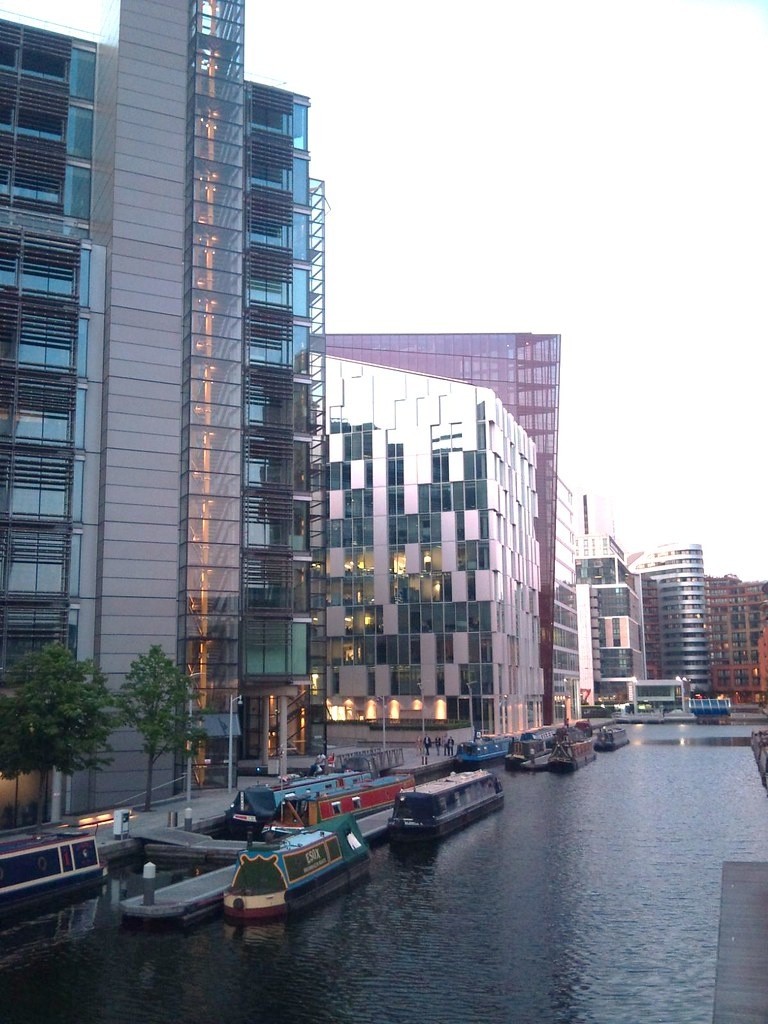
[417, 683, 425, 748]
[187, 673, 206, 802]
[466, 680, 476, 737]
[230, 696, 242, 794]
[377, 696, 386, 753]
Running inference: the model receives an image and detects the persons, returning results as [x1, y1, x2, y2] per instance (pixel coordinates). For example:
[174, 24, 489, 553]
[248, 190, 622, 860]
[435, 736, 441, 756]
[423, 734, 431, 756]
[416, 735, 424, 752]
[566, 718, 570, 727]
[448, 736, 454, 756]
[315, 752, 326, 772]
[443, 736, 449, 756]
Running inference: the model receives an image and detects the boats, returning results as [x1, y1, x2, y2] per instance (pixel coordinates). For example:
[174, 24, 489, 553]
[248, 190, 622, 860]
[222, 810, 374, 922]
[548, 734, 596, 771]
[504, 738, 554, 769]
[521, 720, 593, 747]
[453, 735, 515, 765]
[227, 769, 374, 839]
[0, 829, 113, 919]
[263, 773, 419, 842]
[385, 769, 506, 840]
[594, 727, 630, 750]
